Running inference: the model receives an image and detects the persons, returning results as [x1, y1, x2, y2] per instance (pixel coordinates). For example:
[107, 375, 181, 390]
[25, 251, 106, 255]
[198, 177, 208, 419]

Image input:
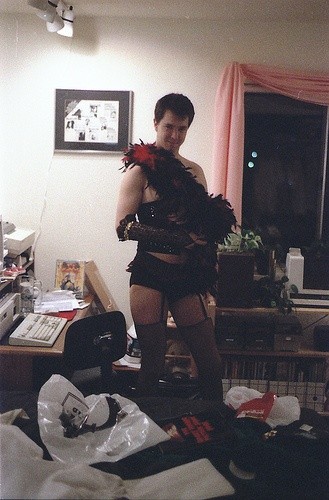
[116, 93, 224, 401]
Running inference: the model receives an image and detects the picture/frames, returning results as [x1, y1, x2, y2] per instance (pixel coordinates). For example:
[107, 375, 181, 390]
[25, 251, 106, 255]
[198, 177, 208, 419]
[56, 89, 132, 153]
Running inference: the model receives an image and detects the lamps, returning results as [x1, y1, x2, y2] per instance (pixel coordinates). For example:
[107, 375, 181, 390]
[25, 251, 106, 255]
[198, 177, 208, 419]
[29, 0, 74, 37]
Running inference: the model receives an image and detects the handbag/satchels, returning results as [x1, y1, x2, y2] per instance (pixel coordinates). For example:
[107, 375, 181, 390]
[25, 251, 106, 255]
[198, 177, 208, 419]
[38, 375, 171, 465]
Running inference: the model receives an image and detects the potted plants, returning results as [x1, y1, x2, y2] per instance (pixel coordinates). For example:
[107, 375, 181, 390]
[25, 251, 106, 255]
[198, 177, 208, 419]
[268, 277, 303, 351]
[211, 227, 262, 308]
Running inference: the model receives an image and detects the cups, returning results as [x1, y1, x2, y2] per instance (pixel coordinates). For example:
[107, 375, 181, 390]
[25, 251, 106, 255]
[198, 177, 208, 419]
[17, 275, 34, 311]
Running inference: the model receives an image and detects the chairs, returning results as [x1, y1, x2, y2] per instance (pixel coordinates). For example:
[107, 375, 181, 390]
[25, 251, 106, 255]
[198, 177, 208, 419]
[62, 310, 127, 399]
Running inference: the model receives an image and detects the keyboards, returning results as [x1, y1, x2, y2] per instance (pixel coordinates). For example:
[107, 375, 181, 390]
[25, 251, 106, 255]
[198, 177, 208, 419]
[11, 312, 68, 347]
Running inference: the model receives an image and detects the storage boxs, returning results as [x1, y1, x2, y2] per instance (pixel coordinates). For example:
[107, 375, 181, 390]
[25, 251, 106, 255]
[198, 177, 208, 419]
[243, 323, 274, 351]
[85, 262, 119, 313]
[215, 316, 246, 351]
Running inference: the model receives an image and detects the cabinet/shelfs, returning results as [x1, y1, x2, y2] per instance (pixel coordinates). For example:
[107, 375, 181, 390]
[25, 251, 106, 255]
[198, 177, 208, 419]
[0, 248, 94, 414]
